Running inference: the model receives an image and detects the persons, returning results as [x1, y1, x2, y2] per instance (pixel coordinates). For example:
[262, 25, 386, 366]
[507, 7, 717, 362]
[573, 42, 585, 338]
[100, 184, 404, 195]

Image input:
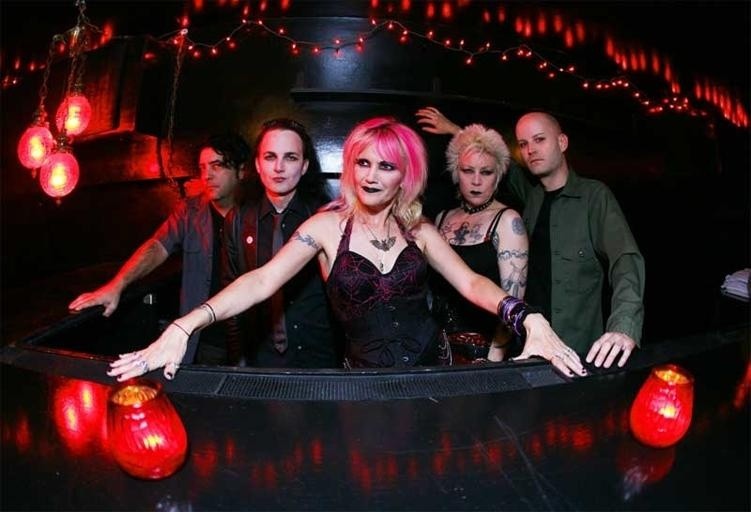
[426, 122, 527, 367]
[414, 105, 647, 368]
[219, 116, 344, 369]
[104, 116, 587, 386]
[68, 134, 256, 365]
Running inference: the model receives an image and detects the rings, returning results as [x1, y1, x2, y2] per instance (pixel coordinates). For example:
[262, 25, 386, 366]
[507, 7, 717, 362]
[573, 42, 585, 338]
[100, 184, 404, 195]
[131, 351, 149, 372]
[549, 346, 574, 362]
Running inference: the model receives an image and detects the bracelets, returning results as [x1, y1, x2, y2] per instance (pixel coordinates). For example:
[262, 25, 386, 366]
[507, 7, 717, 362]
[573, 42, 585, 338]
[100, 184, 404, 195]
[199, 300, 219, 322]
[496, 294, 536, 333]
[170, 321, 191, 337]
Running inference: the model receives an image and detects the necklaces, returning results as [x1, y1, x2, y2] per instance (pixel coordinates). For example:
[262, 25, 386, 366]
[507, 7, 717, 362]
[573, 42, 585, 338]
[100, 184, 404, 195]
[359, 204, 397, 253]
[459, 198, 493, 214]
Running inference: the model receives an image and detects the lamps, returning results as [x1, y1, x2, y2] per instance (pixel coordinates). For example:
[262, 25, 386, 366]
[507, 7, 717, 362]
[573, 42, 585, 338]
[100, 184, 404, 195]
[627, 367, 694, 448]
[16, 0, 101, 205]
[105, 382, 188, 482]
